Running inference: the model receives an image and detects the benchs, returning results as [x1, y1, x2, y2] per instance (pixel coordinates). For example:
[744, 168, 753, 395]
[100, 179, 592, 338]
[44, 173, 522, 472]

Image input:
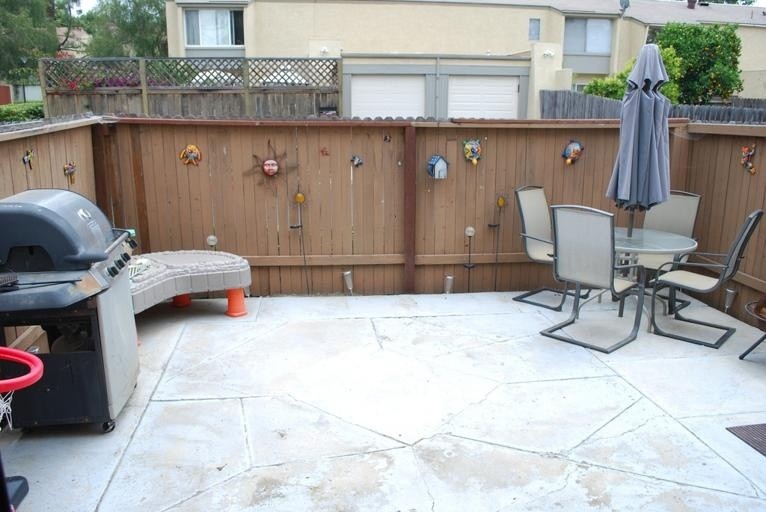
[126, 249, 252, 346]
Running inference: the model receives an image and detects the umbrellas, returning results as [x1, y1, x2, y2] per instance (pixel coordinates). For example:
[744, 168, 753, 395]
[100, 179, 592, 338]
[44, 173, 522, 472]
[600, 39, 673, 318]
